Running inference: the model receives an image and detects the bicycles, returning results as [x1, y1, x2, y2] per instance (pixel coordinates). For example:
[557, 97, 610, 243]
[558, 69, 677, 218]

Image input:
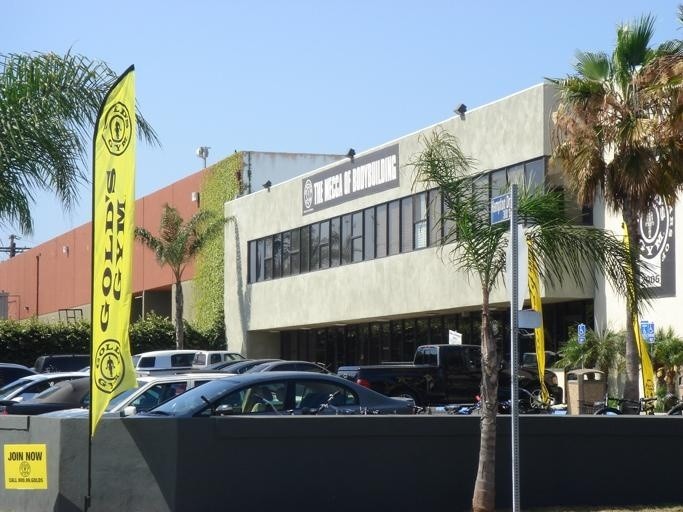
[593, 398, 682, 416]
[200, 391, 476, 415]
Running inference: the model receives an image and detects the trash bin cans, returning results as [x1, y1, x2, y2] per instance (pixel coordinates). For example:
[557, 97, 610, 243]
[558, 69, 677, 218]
[566, 368, 605, 415]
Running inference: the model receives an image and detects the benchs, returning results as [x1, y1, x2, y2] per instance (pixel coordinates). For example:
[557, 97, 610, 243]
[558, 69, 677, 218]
[296, 392, 344, 408]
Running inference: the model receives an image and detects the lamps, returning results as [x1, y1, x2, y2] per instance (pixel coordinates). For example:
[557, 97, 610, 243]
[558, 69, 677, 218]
[343, 149, 354, 160]
[191, 191, 201, 200]
[453, 104, 468, 117]
[61, 245, 71, 257]
[262, 180, 271, 190]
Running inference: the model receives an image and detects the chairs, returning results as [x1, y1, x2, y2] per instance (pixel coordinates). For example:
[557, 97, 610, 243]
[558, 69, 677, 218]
[251, 386, 271, 412]
[273, 386, 288, 412]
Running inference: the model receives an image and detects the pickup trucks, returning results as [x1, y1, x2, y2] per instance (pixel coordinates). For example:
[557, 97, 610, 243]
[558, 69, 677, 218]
[336, 344, 558, 414]
